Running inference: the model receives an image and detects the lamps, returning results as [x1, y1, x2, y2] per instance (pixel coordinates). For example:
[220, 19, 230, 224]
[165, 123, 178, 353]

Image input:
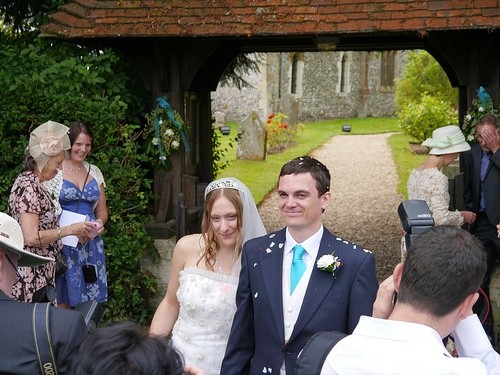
[219, 126, 231, 135]
[342, 125, 352, 132]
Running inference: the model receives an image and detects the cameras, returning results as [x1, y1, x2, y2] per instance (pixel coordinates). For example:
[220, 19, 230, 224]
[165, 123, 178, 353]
[397, 199, 435, 252]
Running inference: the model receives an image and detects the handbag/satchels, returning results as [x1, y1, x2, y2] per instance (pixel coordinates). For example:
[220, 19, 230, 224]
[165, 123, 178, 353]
[32, 284, 57, 304]
[81, 264, 97, 283]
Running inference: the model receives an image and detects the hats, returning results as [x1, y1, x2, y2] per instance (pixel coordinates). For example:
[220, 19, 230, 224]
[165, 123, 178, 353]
[422, 125, 471, 156]
[1, 212, 55, 267]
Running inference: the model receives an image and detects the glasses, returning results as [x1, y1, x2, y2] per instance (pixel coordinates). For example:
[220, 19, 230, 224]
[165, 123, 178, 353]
[4, 252, 21, 287]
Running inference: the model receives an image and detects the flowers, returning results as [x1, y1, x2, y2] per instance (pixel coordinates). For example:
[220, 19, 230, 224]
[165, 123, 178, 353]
[316, 251, 341, 276]
[144, 98, 184, 163]
[462, 87, 498, 143]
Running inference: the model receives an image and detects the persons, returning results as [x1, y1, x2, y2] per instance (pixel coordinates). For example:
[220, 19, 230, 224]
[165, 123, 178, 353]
[7, 119, 97, 304]
[462, 113, 500, 353]
[0, 212, 91, 375]
[66, 323, 206, 375]
[396, 125, 477, 264]
[293, 224, 500, 375]
[218, 155, 380, 375]
[40, 121, 107, 309]
[147, 177, 266, 375]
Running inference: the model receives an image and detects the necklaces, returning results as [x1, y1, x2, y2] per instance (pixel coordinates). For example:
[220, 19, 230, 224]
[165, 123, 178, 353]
[64, 160, 84, 172]
[215, 254, 233, 276]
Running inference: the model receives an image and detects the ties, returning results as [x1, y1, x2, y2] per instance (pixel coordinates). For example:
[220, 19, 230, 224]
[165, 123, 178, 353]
[289, 244, 308, 295]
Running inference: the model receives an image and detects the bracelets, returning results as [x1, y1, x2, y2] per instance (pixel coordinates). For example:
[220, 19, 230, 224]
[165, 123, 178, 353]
[57, 227, 62, 240]
[95, 219, 104, 224]
